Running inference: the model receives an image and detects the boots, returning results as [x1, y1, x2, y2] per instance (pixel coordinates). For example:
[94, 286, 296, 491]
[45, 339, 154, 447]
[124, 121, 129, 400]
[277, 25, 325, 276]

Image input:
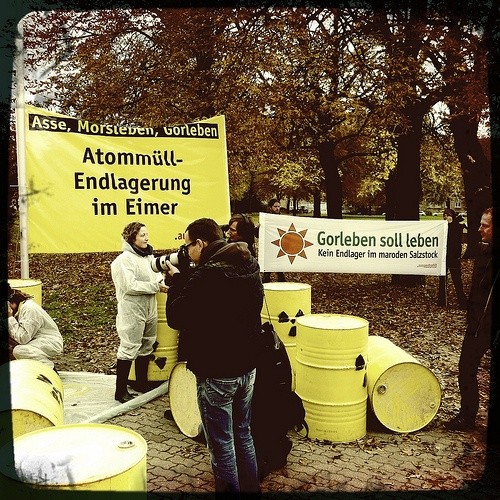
[114, 359, 135, 404]
[133, 355, 158, 393]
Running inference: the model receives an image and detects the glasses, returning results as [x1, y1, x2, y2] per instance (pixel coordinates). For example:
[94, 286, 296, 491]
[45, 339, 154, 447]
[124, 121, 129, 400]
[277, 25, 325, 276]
[185, 241, 195, 250]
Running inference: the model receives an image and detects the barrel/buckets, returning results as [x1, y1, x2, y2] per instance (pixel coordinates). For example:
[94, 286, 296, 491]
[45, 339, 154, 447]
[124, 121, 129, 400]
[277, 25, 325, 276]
[127, 291, 183, 382]
[261, 282, 312, 391]
[0, 424, 148, 500]
[7, 279, 42, 315]
[0, 359, 64, 445]
[367, 336, 441, 434]
[168, 361, 204, 438]
[294, 313, 370, 444]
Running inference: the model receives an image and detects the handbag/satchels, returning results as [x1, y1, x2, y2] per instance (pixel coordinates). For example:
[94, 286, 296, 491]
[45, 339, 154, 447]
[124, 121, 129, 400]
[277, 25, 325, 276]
[252, 323, 292, 405]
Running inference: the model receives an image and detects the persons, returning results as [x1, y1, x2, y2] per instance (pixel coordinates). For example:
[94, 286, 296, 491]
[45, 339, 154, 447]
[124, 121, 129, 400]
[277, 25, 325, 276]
[6, 282, 65, 376]
[224, 213, 256, 257]
[164, 219, 266, 492]
[436, 208, 469, 311]
[252, 199, 290, 282]
[110, 221, 168, 405]
[443, 207, 495, 444]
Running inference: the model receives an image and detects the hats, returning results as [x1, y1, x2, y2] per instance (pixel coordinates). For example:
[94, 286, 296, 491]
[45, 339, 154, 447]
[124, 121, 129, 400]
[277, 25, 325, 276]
[122, 222, 145, 245]
[444, 208, 456, 219]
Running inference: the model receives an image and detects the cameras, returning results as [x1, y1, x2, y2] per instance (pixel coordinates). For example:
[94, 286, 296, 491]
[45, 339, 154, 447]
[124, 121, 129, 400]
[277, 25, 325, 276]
[150, 249, 190, 273]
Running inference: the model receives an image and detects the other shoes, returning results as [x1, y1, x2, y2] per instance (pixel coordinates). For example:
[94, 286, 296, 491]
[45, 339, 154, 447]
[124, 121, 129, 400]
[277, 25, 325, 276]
[443, 414, 475, 431]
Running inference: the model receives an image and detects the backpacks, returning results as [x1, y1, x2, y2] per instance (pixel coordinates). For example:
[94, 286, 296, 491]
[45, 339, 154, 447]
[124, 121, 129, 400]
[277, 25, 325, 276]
[250, 390, 308, 482]
